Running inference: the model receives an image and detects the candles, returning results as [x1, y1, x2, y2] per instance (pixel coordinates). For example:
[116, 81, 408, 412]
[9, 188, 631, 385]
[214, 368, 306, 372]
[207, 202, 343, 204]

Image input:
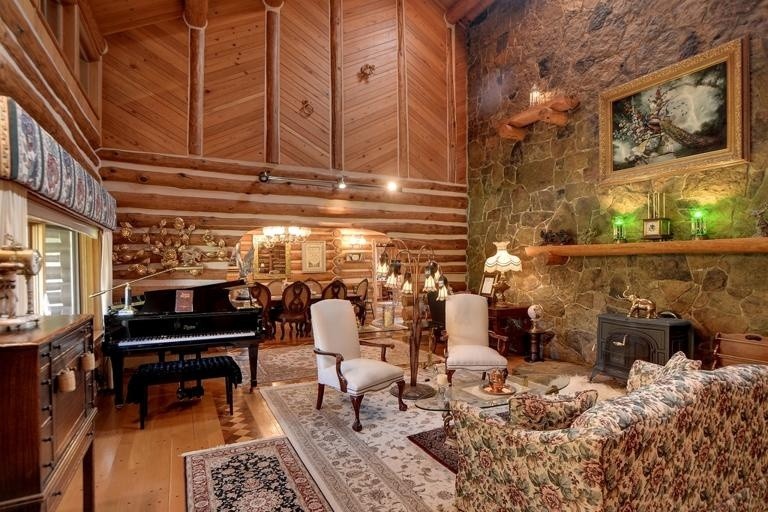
[436, 374, 448, 386]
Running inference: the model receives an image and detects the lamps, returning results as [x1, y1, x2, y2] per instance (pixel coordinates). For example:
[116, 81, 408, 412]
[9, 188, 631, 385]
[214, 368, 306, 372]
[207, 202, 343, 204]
[261, 223, 312, 245]
[89, 264, 204, 316]
[336, 176, 350, 193]
[377, 236, 451, 401]
[689, 206, 711, 240]
[612, 217, 629, 244]
[528, 84, 541, 107]
[484, 239, 522, 308]
[257, 166, 271, 184]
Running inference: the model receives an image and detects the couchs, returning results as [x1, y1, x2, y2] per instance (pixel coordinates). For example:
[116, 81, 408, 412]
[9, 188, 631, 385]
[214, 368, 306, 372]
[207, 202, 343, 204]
[453, 356, 767, 511]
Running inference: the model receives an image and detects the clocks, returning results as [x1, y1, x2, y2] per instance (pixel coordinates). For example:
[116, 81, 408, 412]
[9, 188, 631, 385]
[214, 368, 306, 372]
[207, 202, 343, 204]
[642, 190, 675, 243]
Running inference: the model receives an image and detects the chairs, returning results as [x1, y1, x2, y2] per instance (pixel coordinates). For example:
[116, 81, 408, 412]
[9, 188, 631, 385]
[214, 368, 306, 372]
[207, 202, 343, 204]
[310, 298, 408, 431]
[438, 289, 509, 395]
[246, 276, 371, 342]
[427, 290, 446, 354]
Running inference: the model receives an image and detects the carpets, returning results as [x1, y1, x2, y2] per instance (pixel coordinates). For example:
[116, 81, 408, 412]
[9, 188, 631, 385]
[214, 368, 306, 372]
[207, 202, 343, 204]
[357, 317, 409, 336]
[258, 360, 627, 512]
[222, 337, 447, 391]
[177, 436, 334, 512]
[404, 410, 510, 473]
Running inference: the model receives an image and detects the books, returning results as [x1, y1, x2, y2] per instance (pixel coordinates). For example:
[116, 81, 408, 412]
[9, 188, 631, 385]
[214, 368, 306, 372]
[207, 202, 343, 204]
[174, 289, 194, 312]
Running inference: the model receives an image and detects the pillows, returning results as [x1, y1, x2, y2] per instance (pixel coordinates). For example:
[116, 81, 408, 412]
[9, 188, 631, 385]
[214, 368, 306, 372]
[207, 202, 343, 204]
[506, 389, 598, 430]
[640, 348, 703, 391]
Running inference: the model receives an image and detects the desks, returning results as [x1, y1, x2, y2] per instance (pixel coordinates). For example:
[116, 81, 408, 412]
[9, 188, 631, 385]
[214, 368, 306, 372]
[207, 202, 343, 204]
[488, 304, 531, 356]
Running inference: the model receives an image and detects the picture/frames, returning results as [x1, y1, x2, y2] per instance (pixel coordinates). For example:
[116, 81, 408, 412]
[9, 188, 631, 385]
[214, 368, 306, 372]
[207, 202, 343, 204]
[229, 242, 241, 272]
[478, 272, 500, 297]
[301, 241, 327, 275]
[598, 35, 751, 188]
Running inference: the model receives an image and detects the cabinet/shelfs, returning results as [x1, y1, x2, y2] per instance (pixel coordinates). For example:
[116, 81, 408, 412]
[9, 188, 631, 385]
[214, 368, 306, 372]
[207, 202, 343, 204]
[590, 313, 692, 383]
[0, 316, 95, 512]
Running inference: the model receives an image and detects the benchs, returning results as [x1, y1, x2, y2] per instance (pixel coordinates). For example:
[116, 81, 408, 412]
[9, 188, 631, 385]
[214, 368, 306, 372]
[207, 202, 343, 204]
[133, 356, 240, 430]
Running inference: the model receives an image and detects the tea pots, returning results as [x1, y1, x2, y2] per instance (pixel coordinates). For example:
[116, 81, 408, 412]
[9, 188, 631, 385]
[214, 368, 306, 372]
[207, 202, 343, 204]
[487, 368, 505, 393]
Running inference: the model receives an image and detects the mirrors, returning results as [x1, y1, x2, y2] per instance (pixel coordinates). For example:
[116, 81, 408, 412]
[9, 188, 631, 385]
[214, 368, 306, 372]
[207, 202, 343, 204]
[251, 234, 291, 280]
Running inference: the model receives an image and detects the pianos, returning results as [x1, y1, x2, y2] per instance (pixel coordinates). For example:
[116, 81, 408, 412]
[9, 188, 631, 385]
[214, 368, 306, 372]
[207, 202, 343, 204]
[104, 279, 265, 408]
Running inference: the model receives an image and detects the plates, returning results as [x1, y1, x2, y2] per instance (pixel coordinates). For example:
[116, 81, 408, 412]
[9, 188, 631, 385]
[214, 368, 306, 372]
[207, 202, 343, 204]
[481, 382, 516, 395]
